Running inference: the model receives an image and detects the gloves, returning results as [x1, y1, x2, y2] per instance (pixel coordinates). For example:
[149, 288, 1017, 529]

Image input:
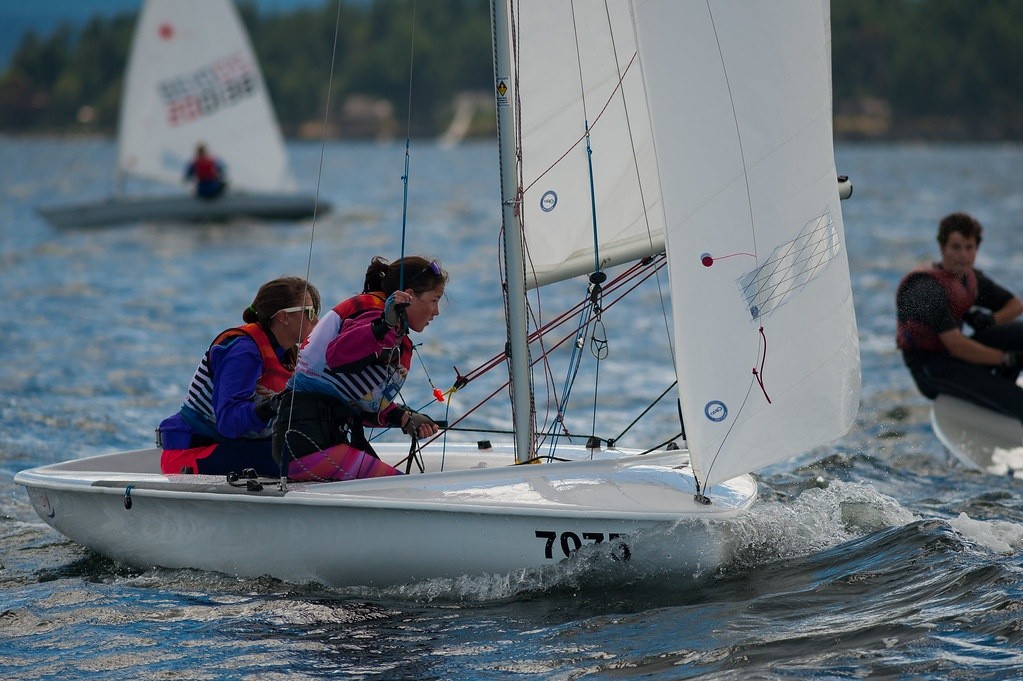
[968, 311, 996, 333]
[1005, 351, 1023, 368]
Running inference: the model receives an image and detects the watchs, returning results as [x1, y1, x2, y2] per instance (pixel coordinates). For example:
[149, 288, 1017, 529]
[1001, 352, 1009, 368]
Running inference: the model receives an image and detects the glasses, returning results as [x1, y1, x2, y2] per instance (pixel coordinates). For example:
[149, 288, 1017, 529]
[270, 306, 316, 322]
[405, 262, 441, 289]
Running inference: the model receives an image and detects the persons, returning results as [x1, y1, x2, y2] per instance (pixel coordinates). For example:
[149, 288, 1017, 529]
[896, 214, 1023, 425]
[275, 256, 445, 482]
[183, 142, 228, 198]
[156, 275, 320, 477]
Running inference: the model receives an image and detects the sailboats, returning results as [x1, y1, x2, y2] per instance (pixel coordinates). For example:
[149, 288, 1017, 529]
[12, 0, 861, 606]
[34, 0, 334, 233]
